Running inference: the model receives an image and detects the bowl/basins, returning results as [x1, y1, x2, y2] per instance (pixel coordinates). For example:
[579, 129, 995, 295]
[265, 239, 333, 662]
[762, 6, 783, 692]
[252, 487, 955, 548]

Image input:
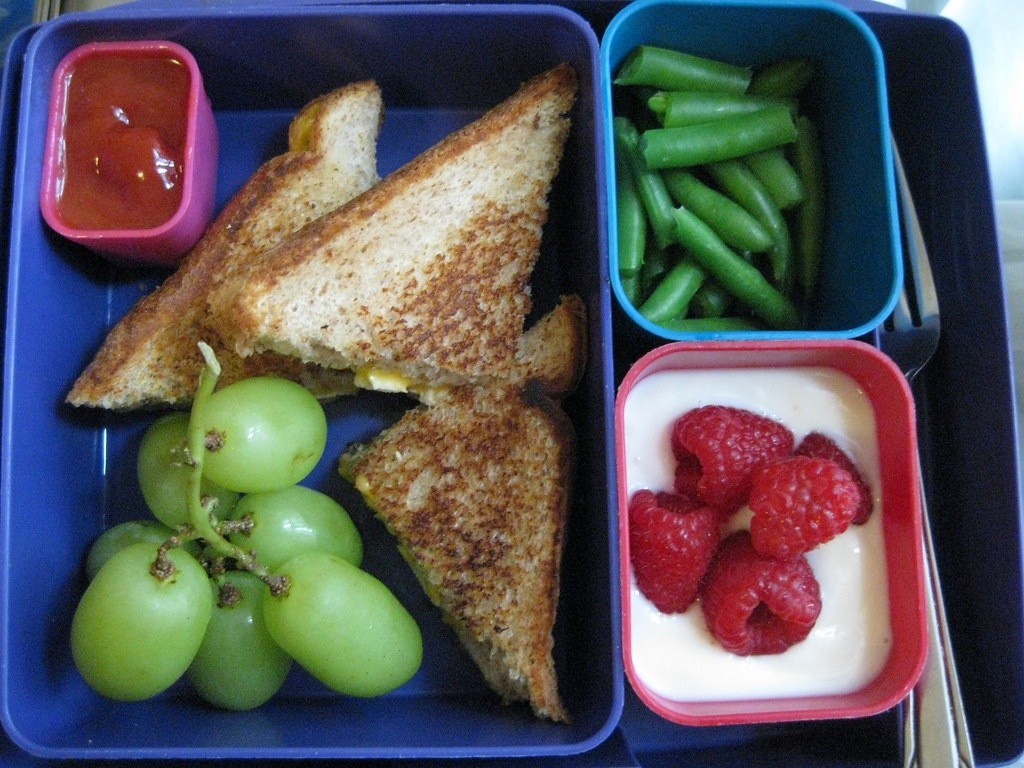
[615, 340, 930, 727]
[598, 0, 907, 343]
[39, 40, 219, 270]
[0, 0, 625, 768]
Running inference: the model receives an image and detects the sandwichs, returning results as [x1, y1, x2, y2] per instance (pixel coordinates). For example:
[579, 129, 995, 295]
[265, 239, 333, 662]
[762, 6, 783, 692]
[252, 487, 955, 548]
[202, 55, 579, 403]
[341, 296, 586, 724]
[62, 81, 380, 409]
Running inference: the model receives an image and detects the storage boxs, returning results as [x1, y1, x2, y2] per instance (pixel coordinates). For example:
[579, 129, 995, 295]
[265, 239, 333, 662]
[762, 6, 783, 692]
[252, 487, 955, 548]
[614, 342, 930, 726]
[40, 38, 219, 261]
[0, 0, 625, 760]
[599, 0, 906, 338]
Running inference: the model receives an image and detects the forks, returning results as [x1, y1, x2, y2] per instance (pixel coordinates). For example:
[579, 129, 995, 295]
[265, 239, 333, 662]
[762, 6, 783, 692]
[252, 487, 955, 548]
[861, 134, 974, 768]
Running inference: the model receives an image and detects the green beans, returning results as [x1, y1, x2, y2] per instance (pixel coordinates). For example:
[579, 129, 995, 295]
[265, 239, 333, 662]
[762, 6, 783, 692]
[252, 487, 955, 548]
[615, 44, 823, 332]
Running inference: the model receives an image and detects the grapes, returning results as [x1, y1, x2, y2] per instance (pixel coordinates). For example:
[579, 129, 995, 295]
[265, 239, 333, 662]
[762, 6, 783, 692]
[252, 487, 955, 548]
[69, 376, 424, 709]
